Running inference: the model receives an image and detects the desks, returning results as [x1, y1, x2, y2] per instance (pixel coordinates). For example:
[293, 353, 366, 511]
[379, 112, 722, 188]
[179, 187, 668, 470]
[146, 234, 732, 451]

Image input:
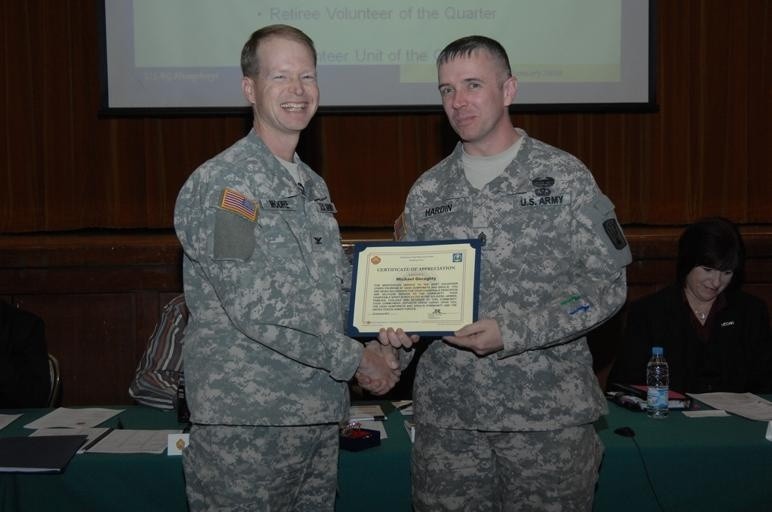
[1, 390, 772, 512]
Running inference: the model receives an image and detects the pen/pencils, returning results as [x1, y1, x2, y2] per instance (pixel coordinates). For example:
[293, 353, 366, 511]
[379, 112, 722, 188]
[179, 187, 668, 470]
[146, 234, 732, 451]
[85, 427, 114, 450]
[399, 403, 412, 410]
[349, 416, 388, 422]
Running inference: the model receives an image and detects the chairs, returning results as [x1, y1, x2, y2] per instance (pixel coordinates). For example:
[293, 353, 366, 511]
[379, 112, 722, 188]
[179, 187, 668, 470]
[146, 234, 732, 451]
[1, 353, 63, 407]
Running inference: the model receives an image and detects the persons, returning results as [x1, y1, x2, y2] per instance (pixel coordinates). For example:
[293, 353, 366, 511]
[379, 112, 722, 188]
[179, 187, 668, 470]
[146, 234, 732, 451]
[128, 291, 190, 411]
[604, 215, 771, 397]
[173, 24, 400, 512]
[378, 35, 632, 512]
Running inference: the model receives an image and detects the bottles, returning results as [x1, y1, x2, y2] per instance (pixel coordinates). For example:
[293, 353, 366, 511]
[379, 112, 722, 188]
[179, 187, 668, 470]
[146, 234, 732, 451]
[645, 348, 670, 419]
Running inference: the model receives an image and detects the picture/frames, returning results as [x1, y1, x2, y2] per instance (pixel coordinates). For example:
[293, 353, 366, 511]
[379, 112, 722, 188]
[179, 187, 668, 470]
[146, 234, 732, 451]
[346, 237, 483, 341]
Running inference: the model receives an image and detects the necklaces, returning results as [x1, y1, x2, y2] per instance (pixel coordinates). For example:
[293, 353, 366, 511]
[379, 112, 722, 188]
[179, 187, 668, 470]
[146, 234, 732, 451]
[686, 294, 712, 321]
